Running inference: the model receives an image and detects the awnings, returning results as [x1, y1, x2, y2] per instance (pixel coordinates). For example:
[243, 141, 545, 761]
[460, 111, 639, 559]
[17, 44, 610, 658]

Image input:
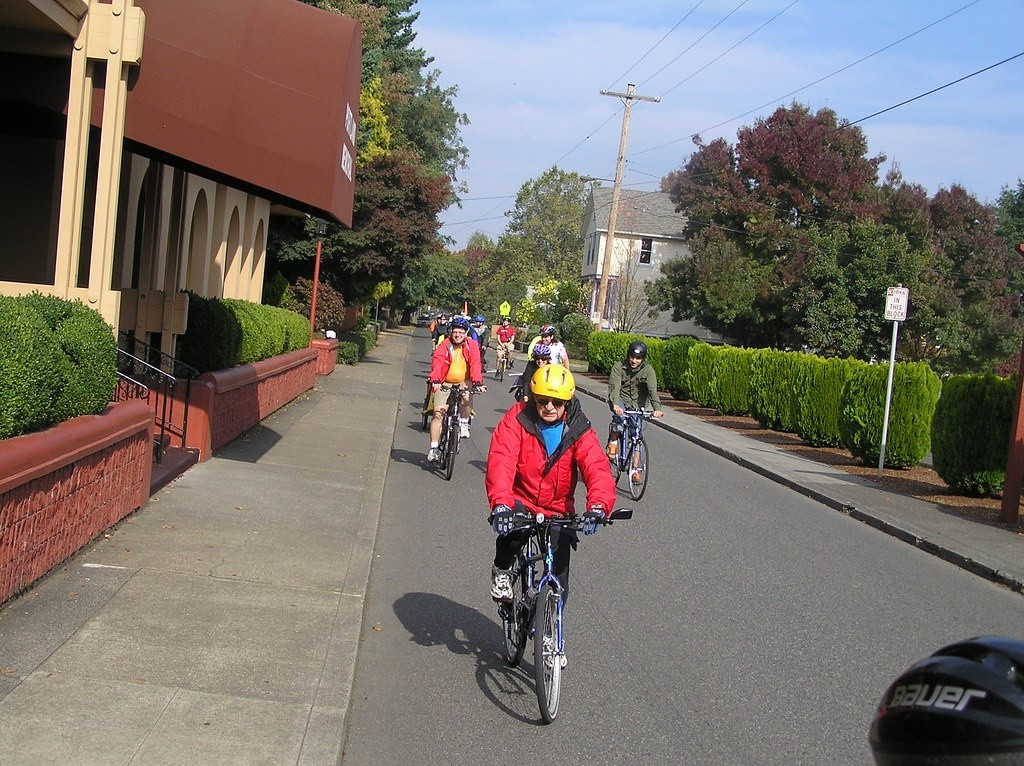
[91, 1, 362, 228]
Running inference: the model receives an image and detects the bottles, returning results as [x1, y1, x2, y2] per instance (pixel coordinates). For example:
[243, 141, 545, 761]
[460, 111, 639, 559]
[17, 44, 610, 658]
[525, 579, 540, 604]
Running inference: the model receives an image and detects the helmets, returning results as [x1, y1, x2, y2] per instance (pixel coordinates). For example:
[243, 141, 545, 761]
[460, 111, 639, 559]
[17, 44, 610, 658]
[531, 345, 551, 358]
[451, 318, 469, 330]
[503, 317, 511, 323]
[475, 315, 484, 322]
[530, 364, 575, 400]
[536, 325, 555, 336]
[629, 341, 647, 357]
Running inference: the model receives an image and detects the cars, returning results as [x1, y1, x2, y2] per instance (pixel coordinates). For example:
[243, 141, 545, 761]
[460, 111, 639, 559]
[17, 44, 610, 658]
[419, 309, 449, 320]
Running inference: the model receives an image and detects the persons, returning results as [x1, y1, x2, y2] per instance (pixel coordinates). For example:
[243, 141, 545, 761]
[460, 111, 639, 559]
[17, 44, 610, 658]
[527, 325, 569, 370]
[430, 314, 489, 373]
[495, 317, 515, 377]
[523, 346, 551, 400]
[607, 341, 663, 484]
[428, 317, 487, 461]
[485, 364, 616, 669]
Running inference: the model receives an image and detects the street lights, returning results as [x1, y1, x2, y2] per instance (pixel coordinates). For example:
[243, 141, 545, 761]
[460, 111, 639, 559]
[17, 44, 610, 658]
[580, 174, 622, 330]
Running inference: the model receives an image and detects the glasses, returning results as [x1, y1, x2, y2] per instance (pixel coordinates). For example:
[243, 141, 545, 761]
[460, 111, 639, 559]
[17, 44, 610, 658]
[535, 356, 548, 360]
[535, 395, 565, 406]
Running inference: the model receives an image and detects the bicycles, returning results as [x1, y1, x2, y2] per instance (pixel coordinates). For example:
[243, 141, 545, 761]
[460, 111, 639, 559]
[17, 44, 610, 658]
[487, 506, 633, 726]
[421, 378, 488, 482]
[605, 409, 664, 501]
[430, 346, 489, 381]
[494, 347, 517, 383]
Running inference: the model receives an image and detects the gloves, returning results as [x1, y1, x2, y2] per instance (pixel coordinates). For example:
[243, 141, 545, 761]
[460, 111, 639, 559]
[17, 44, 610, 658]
[578, 509, 604, 535]
[492, 506, 513, 536]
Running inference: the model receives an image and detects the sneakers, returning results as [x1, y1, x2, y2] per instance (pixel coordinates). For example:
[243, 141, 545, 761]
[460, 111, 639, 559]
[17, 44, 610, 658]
[427, 446, 440, 461]
[491, 564, 514, 600]
[459, 421, 470, 437]
[543, 636, 568, 669]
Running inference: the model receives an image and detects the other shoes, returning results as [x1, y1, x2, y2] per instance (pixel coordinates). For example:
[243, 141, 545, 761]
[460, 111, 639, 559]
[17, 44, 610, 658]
[632, 472, 641, 485]
[605, 440, 617, 459]
[507, 363, 510, 370]
[495, 371, 499, 378]
